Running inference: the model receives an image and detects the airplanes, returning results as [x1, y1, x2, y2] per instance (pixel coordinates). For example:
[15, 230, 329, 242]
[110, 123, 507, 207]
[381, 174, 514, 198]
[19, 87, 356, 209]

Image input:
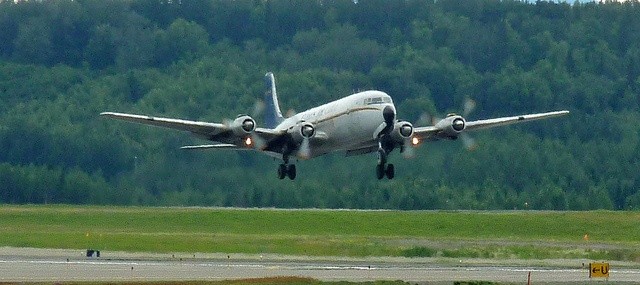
[100, 72, 569, 179]
